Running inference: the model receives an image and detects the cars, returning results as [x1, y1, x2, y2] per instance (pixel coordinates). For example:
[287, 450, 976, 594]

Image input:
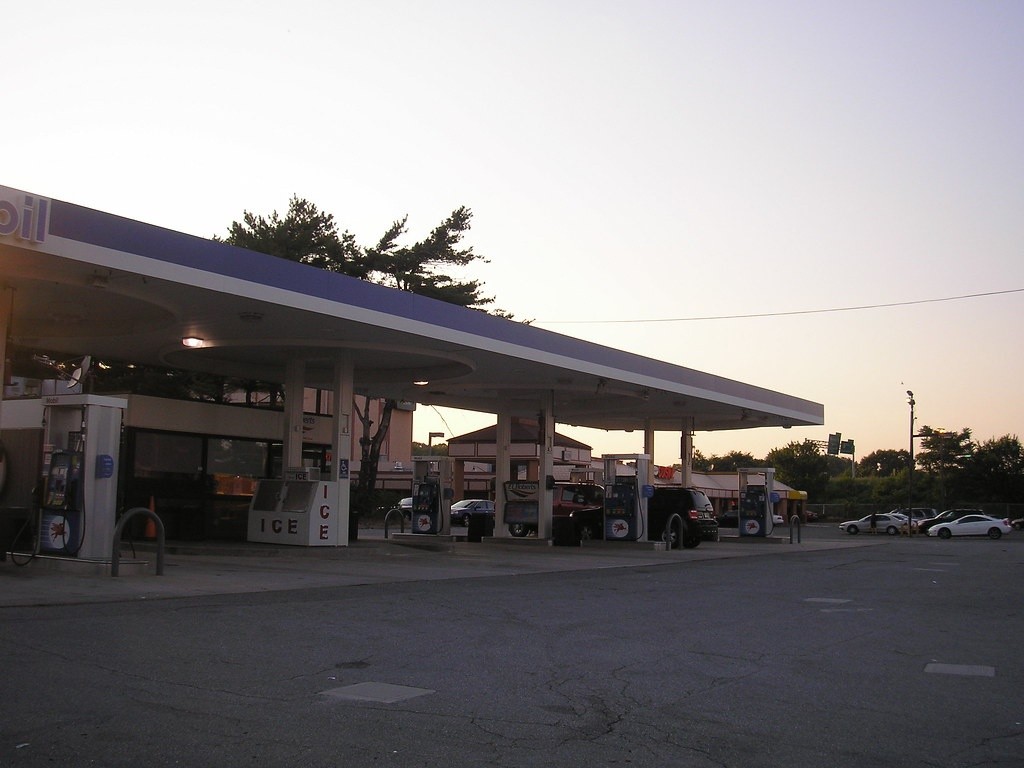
[807, 510, 818, 523]
[773, 514, 784, 526]
[718, 511, 738, 527]
[378, 498, 412, 523]
[451, 499, 495, 526]
[838, 513, 918, 536]
[928, 515, 1012, 539]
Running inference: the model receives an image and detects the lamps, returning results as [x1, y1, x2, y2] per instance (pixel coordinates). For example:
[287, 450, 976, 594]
[90, 272, 110, 289]
[238, 312, 265, 322]
[182, 336, 204, 347]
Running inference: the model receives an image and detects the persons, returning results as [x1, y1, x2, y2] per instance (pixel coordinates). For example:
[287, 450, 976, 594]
[871, 513, 877, 535]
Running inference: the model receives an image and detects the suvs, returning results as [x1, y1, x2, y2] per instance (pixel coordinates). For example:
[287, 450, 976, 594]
[889, 507, 941, 528]
[647, 486, 718, 548]
[917, 509, 985, 536]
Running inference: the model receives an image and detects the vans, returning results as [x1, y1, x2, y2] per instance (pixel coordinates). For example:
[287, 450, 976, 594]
[494, 480, 604, 539]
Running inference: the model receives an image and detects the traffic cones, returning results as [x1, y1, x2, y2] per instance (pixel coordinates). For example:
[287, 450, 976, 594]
[142, 496, 158, 541]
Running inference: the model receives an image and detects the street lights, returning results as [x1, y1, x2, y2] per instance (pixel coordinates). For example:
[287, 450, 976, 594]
[907, 391, 915, 538]
